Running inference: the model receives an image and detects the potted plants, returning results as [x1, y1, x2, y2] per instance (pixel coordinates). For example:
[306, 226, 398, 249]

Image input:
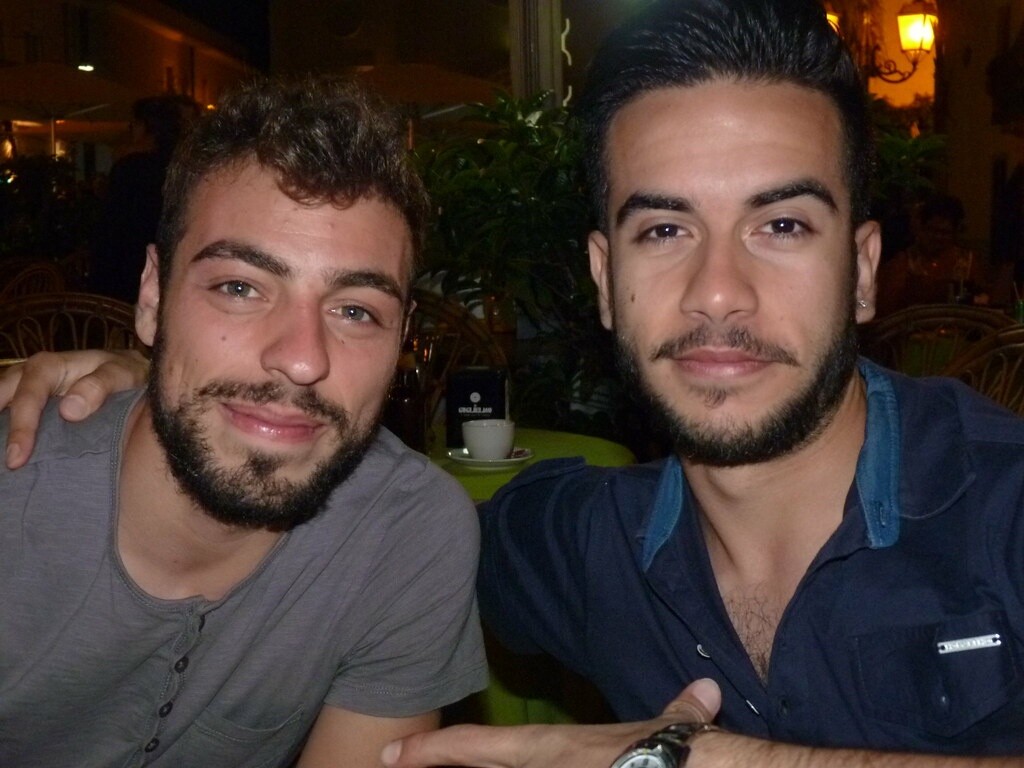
[405, 88, 645, 458]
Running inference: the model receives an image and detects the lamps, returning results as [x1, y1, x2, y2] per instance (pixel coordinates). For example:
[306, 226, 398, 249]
[865, 0, 940, 89]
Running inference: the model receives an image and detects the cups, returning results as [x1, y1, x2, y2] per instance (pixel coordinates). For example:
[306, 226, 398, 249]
[461, 419, 515, 460]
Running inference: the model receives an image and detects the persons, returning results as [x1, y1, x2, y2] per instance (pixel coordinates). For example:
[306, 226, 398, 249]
[0, 68, 489, 768]
[0, 0, 1024, 768]
[989, 165, 1024, 306]
[0, 94, 202, 302]
[871, 195, 980, 320]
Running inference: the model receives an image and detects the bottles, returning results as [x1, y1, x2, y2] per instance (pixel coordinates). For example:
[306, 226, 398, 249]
[382, 341, 426, 455]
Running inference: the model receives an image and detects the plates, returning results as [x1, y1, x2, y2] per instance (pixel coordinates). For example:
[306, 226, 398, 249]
[446, 448, 536, 467]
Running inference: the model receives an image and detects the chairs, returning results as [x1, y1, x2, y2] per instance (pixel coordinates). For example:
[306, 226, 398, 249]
[858, 304, 1023, 416]
[0, 247, 145, 359]
[389, 287, 512, 417]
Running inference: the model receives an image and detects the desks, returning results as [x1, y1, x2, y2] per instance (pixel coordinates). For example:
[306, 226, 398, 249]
[418, 423, 638, 728]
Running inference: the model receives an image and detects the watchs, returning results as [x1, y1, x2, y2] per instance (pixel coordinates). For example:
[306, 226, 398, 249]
[610, 722, 729, 768]
